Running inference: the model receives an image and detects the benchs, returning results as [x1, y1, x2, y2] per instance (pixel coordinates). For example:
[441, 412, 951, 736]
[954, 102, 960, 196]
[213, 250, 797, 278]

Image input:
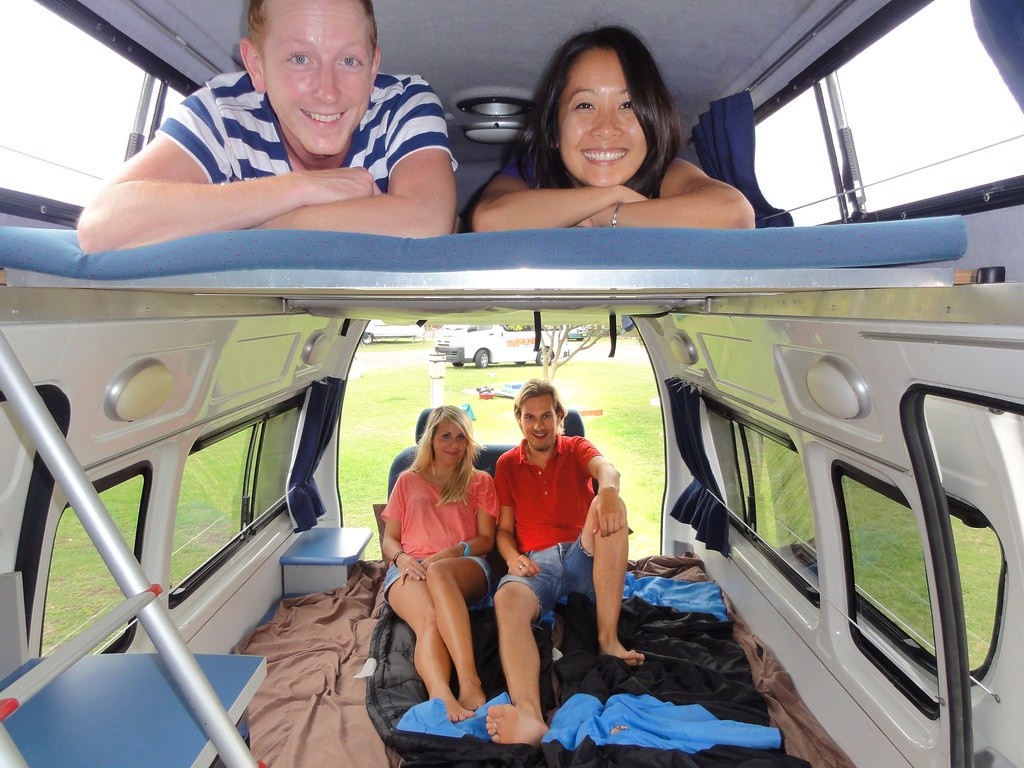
[389, 406, 594, 502]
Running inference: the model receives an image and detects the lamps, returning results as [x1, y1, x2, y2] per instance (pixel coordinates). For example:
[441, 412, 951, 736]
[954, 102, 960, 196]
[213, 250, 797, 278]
[105, 356, 176, 423]
[669, 330, 698, 367]
[462, 122, 527, 144]
[806, 356, 873, 423]
[301, 329, 331, 364]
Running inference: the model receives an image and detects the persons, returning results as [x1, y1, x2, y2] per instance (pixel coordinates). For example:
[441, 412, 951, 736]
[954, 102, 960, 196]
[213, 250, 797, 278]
[486, 377, 646, 745]
[381, 404, 500, 727]
[471, 24, 756, 234]
[74, 0, 462, 258]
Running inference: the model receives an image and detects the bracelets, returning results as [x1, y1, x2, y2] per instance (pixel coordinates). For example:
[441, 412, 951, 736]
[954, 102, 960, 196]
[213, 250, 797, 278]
[394, 552, 405, 567]
[392, 551, 402, 564]
[612, 201, 623, 228]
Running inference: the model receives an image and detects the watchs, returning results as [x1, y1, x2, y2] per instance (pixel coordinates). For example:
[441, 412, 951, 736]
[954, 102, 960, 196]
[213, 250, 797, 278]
[458, 542, 470, 556]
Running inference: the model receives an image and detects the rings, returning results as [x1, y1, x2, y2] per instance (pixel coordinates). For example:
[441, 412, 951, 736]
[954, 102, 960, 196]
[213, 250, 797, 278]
[406, 568, 409, 572]
[520, 564, 525, 570]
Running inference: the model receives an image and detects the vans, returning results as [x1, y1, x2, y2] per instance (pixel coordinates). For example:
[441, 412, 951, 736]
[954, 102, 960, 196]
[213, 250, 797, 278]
[434, 325, 571, 369]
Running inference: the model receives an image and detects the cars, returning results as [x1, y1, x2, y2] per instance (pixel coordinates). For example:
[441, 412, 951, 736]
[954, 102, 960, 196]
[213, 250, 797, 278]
[563, 325, 588, 341]
[588, 325, 608, 336]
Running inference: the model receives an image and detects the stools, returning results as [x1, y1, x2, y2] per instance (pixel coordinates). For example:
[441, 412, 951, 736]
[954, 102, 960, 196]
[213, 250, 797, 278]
[280, 525, 375, 594]
[0, 651, 270, 768]
